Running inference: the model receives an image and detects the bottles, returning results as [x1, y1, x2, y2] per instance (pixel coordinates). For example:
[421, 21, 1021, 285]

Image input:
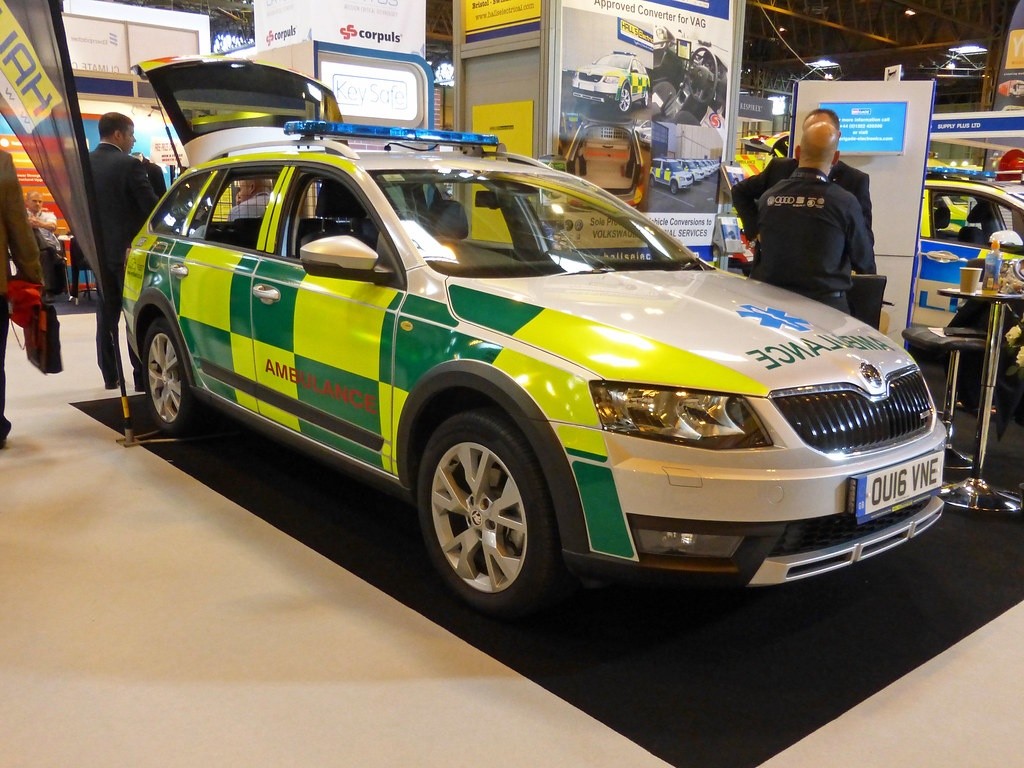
[982, 240, 1003, 296]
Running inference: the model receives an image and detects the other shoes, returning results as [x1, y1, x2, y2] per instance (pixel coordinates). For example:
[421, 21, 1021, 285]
[135, 382, 145, 391]
[105, 377, 124, 389]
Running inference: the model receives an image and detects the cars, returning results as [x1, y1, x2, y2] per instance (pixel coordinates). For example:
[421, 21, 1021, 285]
[741, 130, 1024, 350]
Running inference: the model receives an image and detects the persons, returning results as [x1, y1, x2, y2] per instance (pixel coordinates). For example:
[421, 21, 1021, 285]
[732, 109, 877, 320]
[0, 151, 46, 450]
[227, 177, 274, 222]
[23, 191, 61, 303]
[87, 112, 159, 392]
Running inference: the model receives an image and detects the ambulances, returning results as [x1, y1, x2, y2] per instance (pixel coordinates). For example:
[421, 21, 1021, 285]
[123, 54, 947, 621]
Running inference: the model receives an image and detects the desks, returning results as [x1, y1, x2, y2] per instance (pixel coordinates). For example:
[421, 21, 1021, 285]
[937, 288, 1024, 513]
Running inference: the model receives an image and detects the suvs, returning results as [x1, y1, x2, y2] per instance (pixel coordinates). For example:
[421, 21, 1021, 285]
[571, 52, 651, 115]
[650, 157, 720, 196]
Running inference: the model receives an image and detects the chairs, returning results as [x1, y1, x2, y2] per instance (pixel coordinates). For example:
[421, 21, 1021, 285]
[297, 179, 377, 261]
[429, 200, 470, 240]
[958, 203, 986, 246]
[934, 207, 951, 239]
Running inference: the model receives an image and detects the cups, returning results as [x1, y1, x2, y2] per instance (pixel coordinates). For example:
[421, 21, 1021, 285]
[959, 267, 983, 294]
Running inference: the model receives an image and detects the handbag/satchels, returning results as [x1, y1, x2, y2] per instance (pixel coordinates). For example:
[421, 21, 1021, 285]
[24, 304, 63, 375]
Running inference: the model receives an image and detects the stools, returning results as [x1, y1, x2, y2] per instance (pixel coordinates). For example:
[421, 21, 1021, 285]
[901, 328, 986, 470]
